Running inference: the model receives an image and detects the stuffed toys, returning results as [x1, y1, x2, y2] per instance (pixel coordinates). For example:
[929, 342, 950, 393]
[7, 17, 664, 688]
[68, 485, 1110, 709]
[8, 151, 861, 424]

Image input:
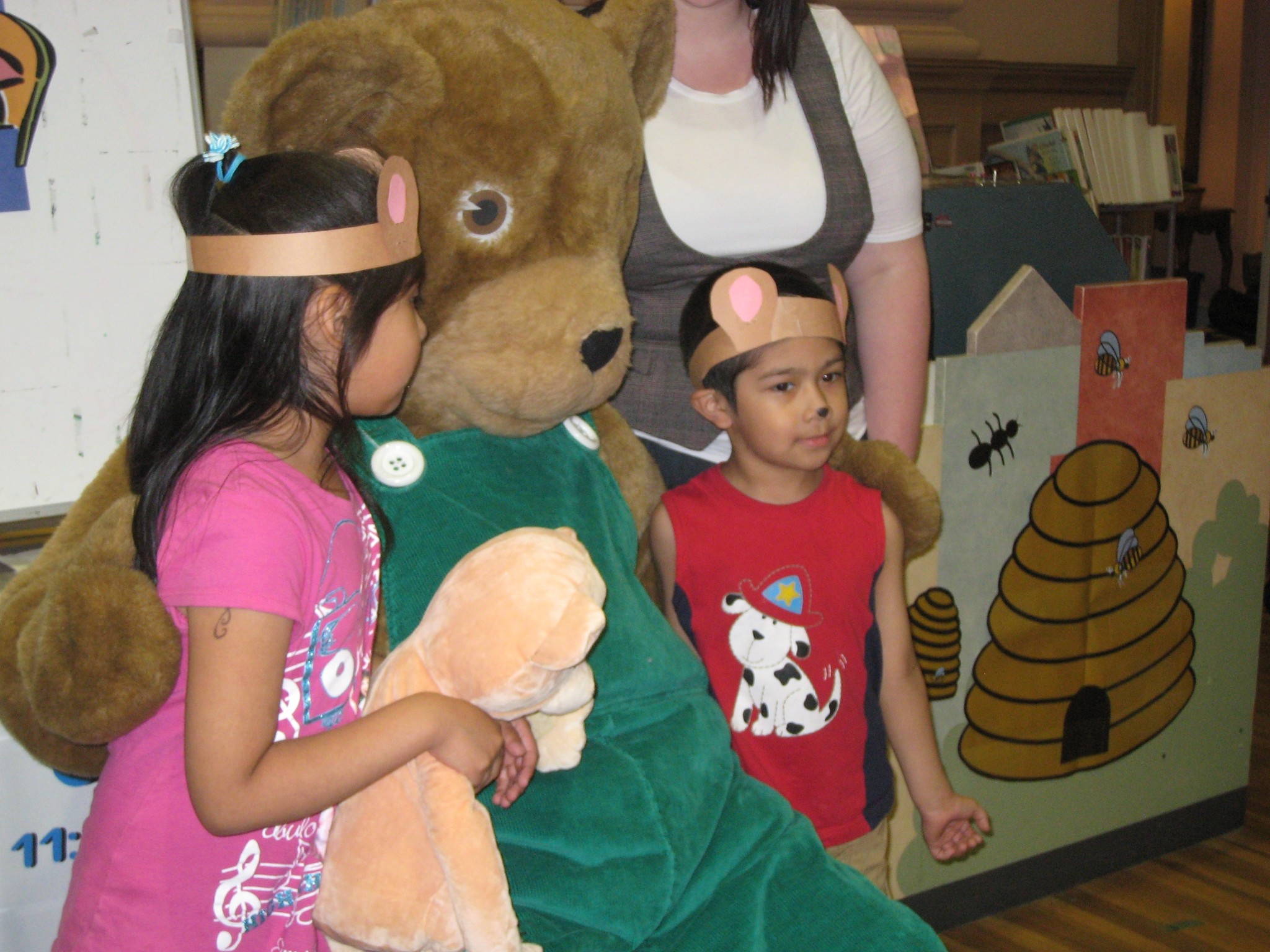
[2, 0, 952, 952]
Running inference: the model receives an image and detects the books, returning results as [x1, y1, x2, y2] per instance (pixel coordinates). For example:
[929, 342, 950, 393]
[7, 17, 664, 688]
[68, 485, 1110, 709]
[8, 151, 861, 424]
[991, 105, 1190, 278]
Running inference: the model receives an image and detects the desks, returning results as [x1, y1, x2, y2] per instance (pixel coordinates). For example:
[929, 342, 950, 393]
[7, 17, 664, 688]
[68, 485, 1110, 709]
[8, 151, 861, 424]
[1156, 206, 1235, 291]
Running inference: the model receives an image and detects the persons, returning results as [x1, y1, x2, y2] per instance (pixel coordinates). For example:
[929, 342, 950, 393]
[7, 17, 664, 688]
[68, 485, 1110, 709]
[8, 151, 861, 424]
[592, 0, 990, 909]
[42, 129, 535, 952]
